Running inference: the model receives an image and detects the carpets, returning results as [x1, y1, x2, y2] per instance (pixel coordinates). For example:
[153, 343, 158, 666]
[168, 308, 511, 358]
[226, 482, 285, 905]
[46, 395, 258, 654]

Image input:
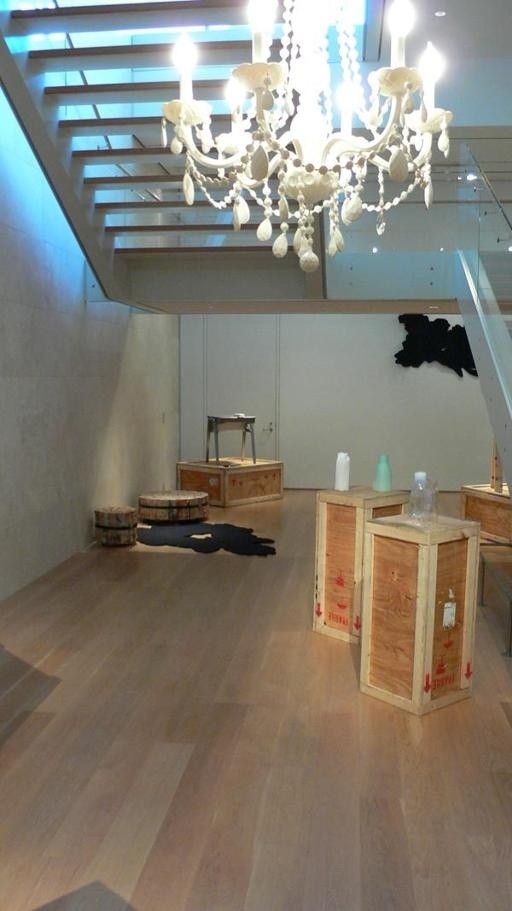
[137, 520, 277, 557]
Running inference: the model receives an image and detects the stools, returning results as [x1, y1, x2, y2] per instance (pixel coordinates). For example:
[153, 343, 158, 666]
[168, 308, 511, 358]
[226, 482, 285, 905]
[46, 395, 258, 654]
[205, 415, 256, 464]
[138, 490, 208, 521]
[93, 505, 138, 546]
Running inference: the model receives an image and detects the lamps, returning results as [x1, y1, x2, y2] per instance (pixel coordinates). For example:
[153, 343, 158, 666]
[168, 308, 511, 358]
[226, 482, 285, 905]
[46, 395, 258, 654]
[162, 0, 454, 273]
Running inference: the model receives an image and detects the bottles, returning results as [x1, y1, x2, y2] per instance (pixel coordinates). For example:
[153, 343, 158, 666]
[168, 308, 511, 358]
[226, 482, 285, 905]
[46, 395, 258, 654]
[406, 470, 436, 532]
[333, 451, 351, 493]
[373, 453, 393, 494]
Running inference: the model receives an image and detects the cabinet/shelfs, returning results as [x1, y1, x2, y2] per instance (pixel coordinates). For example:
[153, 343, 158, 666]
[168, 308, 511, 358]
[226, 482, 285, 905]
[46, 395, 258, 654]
[177, 456, 284, 507]
[360, 512, 481, 716]
[461, 483, 512, 605]
[312, 484, 410, 644]
